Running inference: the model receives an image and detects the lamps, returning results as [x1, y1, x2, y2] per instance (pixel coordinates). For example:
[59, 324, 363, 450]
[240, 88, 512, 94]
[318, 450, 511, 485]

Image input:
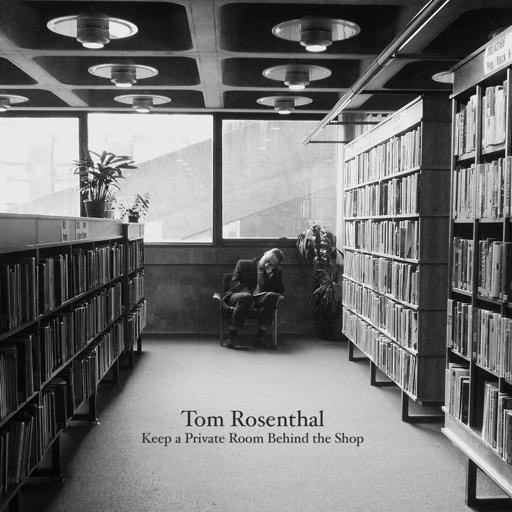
[43, 11, 363, 117]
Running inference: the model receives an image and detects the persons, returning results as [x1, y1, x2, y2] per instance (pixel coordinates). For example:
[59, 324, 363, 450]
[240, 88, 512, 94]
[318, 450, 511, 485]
[222, 247, 285, 350]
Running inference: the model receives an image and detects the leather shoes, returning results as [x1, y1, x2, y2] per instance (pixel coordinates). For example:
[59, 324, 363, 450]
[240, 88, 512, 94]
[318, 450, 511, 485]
[253, 334, 279, 350]
[227, 336, 248, 349]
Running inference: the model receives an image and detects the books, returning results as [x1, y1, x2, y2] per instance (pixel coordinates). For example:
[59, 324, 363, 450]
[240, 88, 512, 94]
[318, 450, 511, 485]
[253, 291, 266, 303]
[1, 241, 148, 501]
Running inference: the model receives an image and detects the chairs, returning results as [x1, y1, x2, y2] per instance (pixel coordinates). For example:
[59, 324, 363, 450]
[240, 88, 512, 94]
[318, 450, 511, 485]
[212, 272, 285, 349]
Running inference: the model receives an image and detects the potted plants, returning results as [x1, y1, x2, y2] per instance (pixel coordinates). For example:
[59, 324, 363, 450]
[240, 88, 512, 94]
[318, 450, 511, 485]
[294, 218, 344, 342]
[74, 144, 151, 222]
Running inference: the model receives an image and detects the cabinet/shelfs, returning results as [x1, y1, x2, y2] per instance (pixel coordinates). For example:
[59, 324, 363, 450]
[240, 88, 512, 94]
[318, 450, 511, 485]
[0, 213, 147, 511]
[340, 25, 511, 512]
